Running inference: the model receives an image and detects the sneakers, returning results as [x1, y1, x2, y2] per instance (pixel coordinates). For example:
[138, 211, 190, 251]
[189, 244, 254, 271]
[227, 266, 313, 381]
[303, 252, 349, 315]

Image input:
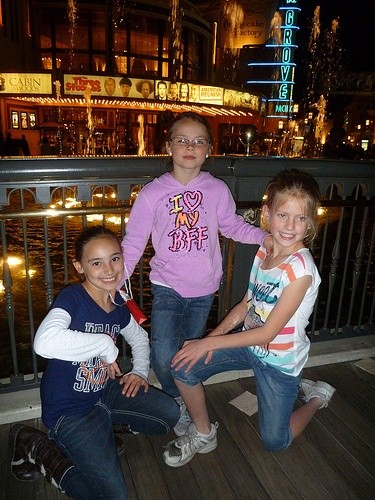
[300, 378, 336, 410]
[173, 396, 193, 435]
[162, 421, 220, 467]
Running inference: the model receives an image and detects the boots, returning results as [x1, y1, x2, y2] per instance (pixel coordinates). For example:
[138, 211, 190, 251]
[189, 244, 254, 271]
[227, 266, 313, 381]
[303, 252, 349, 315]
[113, 423, 139, 455]
[6, 424, 75, 494]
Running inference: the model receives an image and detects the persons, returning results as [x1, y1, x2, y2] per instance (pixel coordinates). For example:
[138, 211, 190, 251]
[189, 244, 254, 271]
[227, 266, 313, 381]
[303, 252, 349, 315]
[168, 82, 180, 102]
[8, 225, 181, 500]
[163, 171, 336, 468]
[115, 112, 274, 437]
[179, 83, 189, 102]
[154, 81, 168, 100]
[136, 80, 154, 98]
[119, 78, 132, 97]
[104, 78, 115, 96]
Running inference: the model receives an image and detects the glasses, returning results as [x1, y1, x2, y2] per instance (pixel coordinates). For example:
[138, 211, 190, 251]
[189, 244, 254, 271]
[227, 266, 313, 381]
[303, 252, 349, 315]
[168, 136, 212, 148]
